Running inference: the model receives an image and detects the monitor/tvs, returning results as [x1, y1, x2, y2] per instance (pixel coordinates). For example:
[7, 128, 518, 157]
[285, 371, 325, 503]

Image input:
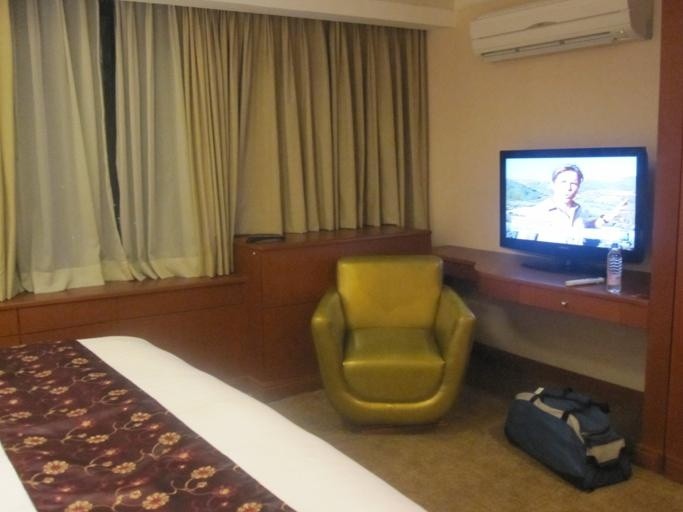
[499, 146, 647, 275]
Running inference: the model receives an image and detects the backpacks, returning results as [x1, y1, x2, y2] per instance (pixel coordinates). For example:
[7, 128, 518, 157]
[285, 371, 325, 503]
[501, 383, 634, 494]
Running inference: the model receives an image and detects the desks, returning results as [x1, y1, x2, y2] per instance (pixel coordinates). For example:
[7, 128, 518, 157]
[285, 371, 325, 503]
[436, 245, 648, 328]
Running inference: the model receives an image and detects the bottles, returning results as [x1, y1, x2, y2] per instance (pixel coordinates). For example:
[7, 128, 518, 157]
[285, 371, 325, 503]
[606, 242, 623, 294]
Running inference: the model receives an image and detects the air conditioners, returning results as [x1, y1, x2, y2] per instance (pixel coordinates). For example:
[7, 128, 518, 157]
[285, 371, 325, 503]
[467, 1, 654, 65]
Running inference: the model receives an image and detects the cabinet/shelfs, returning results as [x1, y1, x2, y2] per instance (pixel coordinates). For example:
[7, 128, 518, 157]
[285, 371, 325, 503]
[231, 224, 432, 404]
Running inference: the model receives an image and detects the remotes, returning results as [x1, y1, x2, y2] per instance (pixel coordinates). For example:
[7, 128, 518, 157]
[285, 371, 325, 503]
[566, 277, 604, 286]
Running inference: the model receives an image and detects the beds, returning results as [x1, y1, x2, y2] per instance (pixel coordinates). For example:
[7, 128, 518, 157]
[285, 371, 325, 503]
[0, 333, 423, 510]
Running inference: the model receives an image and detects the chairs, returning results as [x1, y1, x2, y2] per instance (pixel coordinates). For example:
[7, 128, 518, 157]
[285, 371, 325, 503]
[309, 253, 476, 430]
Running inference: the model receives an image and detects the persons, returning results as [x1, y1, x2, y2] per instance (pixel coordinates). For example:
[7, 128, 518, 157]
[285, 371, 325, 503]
[515, 162, 632, 246]
